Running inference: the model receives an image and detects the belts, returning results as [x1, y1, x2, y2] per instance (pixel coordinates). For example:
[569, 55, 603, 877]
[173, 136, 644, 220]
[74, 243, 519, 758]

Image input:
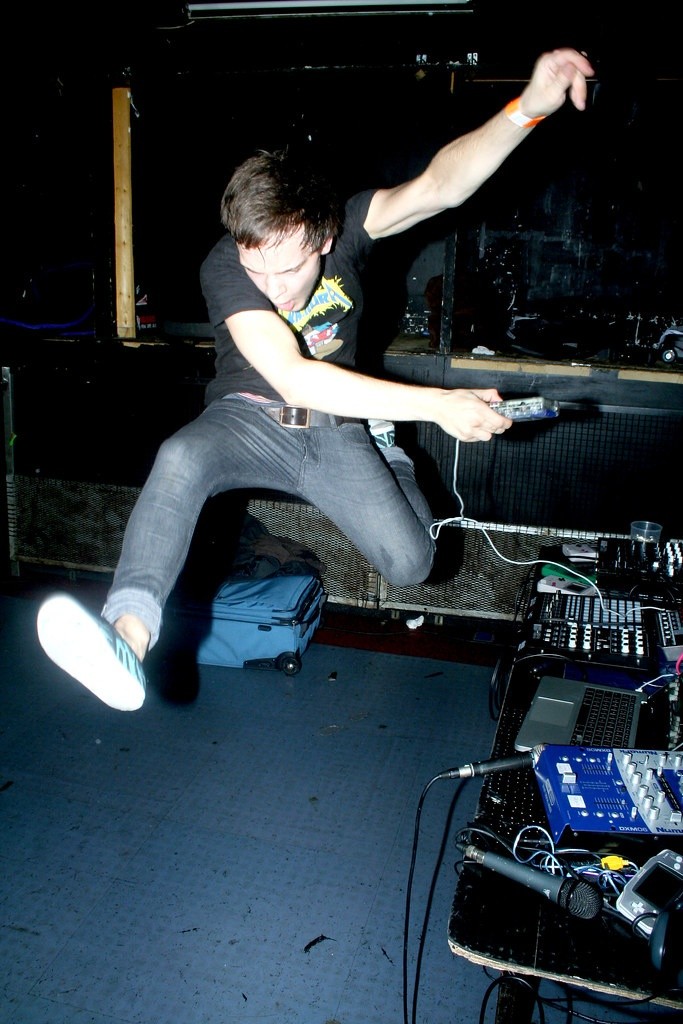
[260, 404, 346, 428]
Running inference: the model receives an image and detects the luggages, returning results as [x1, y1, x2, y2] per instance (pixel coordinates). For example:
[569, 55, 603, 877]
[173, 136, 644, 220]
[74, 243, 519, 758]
[154, 574, 330, 676]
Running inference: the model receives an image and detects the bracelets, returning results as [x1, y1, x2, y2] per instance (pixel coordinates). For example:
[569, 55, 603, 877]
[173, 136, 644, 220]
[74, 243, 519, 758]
[504, 98, 545, 129]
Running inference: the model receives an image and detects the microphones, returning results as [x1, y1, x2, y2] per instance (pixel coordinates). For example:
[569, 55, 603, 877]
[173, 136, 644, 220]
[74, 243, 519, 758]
[456, 843, 601, 919]
[437, 743, 549, 779]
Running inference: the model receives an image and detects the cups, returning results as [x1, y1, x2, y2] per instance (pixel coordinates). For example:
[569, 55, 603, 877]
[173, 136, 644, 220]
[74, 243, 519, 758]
[631, 520, 662, 543]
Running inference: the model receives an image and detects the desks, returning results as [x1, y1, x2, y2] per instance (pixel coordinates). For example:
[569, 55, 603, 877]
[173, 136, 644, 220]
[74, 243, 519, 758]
[446, 545, 683, 1024]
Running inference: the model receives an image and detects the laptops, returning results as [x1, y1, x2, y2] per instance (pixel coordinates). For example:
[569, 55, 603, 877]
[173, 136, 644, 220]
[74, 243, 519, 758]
[515, 671, 683, 752]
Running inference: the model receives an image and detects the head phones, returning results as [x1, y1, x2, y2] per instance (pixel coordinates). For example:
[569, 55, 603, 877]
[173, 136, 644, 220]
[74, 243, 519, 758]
[649, 891, 683, 991]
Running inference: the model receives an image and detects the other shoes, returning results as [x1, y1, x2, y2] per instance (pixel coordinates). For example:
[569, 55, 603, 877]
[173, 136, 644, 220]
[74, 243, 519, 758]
[369, 418, 395, 448]
[37, 592, 147, 711]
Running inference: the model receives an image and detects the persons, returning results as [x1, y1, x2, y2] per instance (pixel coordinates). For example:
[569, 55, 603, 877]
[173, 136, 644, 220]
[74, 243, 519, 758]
[35, 49, 594, 711]
